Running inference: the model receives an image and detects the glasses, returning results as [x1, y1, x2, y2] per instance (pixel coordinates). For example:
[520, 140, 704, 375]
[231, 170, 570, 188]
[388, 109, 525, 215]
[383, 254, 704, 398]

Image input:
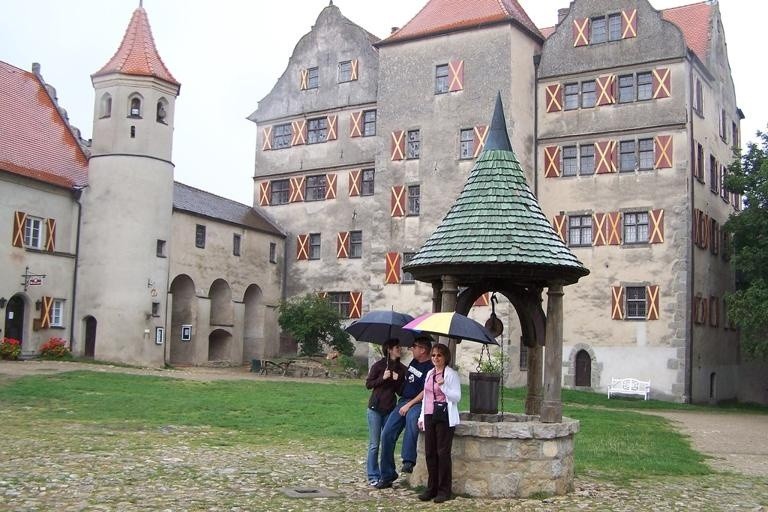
[432, 354, 441, 357]
[412, 344, 422, 348]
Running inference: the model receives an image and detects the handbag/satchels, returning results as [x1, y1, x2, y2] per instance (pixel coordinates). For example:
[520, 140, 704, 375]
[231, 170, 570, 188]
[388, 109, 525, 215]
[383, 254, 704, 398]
[432, 401, 447, 424]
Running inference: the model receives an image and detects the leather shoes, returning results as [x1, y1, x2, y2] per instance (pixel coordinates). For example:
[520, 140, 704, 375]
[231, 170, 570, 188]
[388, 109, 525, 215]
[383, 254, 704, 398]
[433, 494, 449, 503]
[418, 494, 432, 500]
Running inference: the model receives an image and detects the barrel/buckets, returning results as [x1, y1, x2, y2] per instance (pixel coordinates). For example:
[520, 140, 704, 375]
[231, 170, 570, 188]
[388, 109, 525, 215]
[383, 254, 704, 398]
[468, 372, 500, 414]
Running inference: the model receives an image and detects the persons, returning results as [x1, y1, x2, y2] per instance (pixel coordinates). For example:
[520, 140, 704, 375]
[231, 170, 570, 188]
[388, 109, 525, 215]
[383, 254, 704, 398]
[375, 338, 434, 489]
[417, 343, 461, 503]
[366, 338, 407, 486]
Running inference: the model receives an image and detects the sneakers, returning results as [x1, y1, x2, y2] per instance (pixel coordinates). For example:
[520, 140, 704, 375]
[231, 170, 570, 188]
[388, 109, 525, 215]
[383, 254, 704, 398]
[376, 471, 397, 489]
[401, 462, 414, 473]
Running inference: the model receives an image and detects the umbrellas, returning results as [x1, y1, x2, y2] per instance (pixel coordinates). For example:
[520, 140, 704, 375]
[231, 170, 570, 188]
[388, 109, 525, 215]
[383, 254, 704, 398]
[344, 304, 436, 381]
[402, 310, 501, 383]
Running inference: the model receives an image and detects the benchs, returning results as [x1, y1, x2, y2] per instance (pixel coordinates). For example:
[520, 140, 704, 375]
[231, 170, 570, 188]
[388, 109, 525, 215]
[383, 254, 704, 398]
[607, 375, 652, 400]
